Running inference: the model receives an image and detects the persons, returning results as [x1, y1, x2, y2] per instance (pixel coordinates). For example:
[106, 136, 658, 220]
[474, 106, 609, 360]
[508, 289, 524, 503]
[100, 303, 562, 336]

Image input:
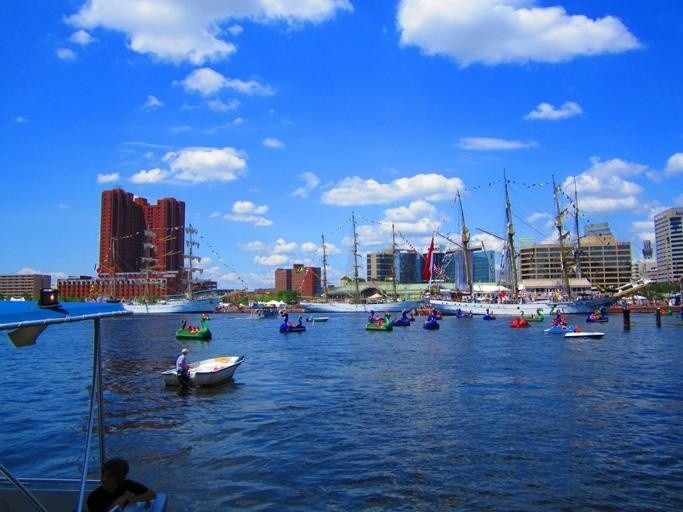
[86, 456, 157, 511]
[175, 347, 192, 396]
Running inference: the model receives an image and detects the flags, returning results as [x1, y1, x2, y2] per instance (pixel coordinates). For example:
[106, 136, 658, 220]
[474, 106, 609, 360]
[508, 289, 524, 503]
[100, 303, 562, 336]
[423, 234, 435, 281]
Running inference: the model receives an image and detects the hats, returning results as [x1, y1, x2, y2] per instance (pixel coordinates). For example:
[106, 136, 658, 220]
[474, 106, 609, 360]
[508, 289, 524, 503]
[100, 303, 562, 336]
[181, 348, 189, 353]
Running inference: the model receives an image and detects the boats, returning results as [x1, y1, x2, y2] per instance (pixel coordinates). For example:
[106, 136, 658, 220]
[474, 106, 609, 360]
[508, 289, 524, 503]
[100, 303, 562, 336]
[125, 297, 221, 313]
[1, 288, 167, 511]
[609, 300, 682, 313]
[429, 288, 620, 316]
[299, 299, 422, 314]
[160, 356, 246, 387]
